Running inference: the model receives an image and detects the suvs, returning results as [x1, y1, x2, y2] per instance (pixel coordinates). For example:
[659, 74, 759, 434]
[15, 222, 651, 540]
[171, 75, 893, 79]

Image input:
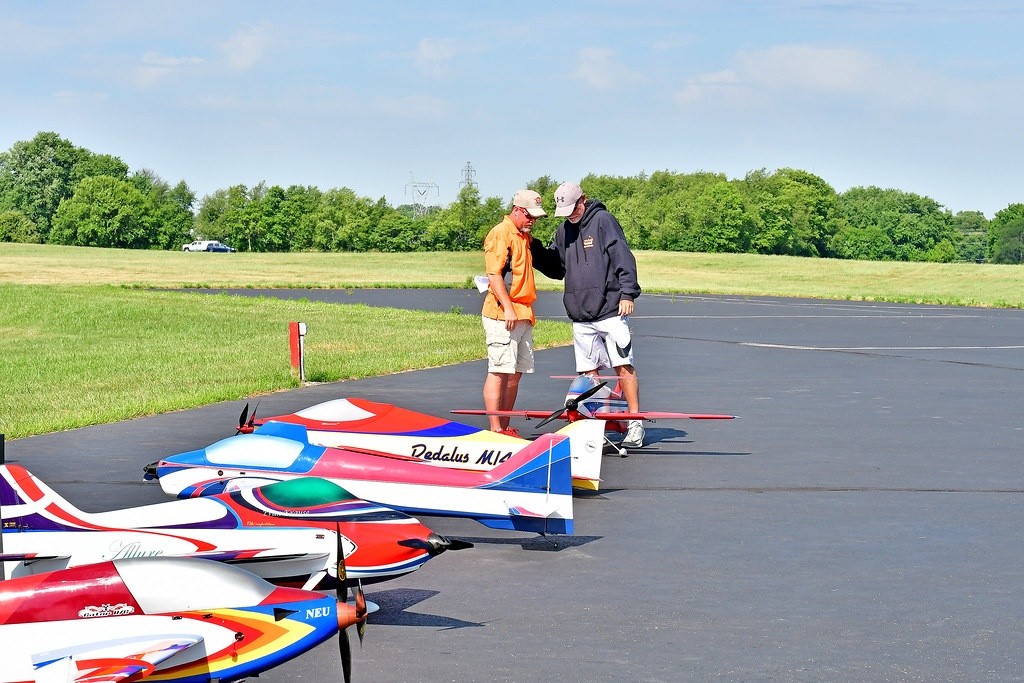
[183, 241, 238, 253]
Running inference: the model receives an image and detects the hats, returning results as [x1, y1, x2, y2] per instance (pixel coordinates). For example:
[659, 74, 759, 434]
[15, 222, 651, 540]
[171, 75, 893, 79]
[513, 190, 548, 218]
[554, 182, 583, 217]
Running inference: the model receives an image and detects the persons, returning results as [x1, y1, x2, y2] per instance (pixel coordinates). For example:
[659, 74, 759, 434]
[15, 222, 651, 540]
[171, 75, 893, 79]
[529, 182, 646, 448]
[482, 190, 549, 439]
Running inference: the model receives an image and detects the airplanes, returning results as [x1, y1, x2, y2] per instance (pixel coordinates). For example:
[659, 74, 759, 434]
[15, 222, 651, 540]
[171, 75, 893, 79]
[0, 397, 607, 683]
[449, 372, 742, 458]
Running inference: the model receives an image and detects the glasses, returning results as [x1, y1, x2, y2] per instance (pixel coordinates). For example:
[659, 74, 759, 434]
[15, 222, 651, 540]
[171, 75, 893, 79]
[574, 198, 581, 211]
[515, 206, 532, 219]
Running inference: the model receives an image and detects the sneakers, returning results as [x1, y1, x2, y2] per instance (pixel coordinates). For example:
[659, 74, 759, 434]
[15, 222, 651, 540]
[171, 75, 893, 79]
[493, 427, 524, 439]
[620, 421, 646, 449]
